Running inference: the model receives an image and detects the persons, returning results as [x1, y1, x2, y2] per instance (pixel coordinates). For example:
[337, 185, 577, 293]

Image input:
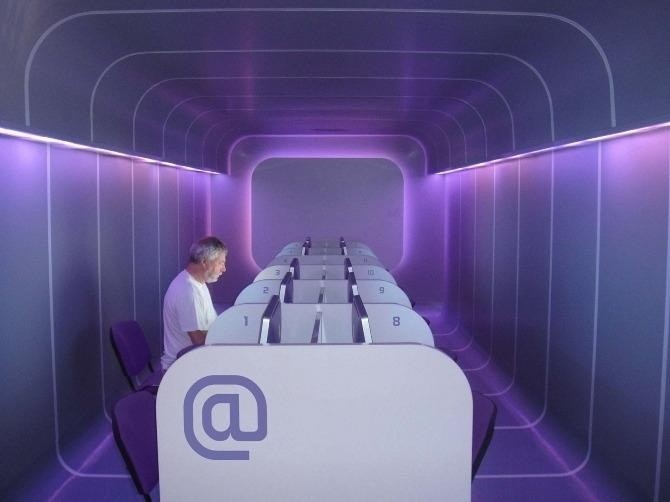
[159, 234, 229, 374]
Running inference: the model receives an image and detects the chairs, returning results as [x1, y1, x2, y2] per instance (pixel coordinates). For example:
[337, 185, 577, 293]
[472, 388, 497, 478]
[110, 320, 167, 502]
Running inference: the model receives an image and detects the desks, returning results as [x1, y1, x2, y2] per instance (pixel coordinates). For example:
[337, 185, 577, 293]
[155, 235, 473, 501]
